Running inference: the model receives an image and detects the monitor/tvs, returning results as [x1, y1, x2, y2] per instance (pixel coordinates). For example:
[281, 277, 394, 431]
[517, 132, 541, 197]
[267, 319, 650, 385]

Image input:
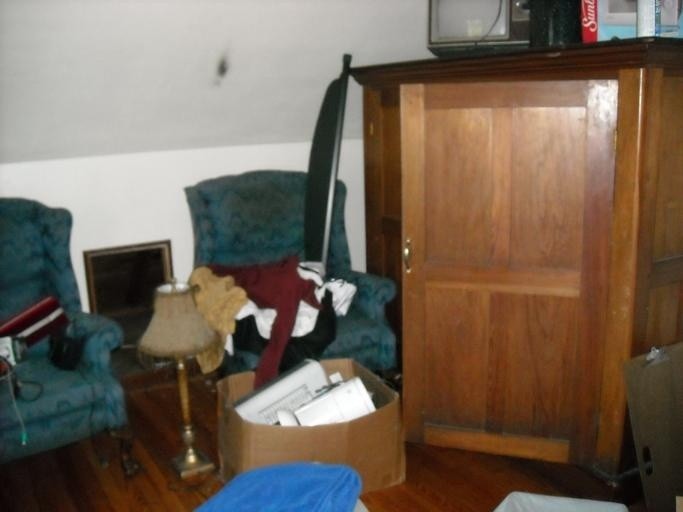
[427, 0, 531, 58]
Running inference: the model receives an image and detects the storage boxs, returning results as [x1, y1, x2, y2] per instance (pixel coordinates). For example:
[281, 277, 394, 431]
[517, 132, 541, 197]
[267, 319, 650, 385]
[213, 356, 406, 496]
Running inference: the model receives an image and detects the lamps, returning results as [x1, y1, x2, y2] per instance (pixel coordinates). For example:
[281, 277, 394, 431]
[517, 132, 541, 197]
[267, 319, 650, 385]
[138, 283, 221, 478]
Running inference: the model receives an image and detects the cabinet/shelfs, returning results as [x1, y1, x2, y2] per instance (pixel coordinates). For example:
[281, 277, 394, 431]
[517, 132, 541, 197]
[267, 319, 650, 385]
[348, 35, 682, 487]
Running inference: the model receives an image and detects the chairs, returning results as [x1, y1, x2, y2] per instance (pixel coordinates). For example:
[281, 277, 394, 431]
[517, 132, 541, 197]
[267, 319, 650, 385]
[2, 199, 124, 467]
[186, 171, 395, 381]
